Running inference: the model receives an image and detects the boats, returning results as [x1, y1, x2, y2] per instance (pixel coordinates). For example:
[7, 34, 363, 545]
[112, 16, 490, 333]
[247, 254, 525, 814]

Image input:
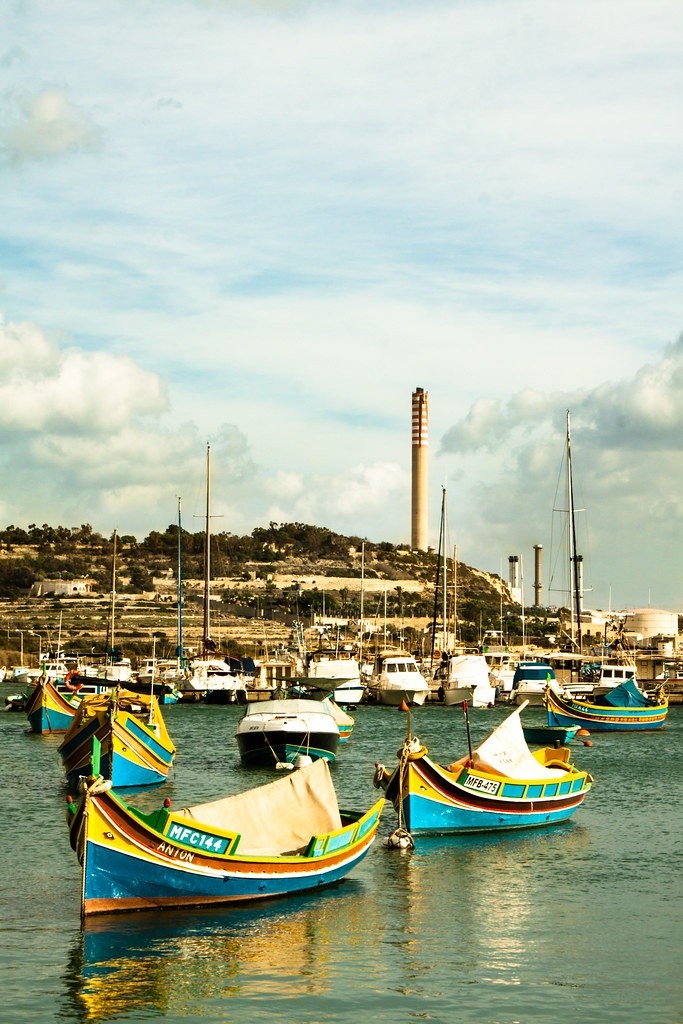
[0, 418, 663, 747]
[371, 699, 594, 839]
[70, 737, 391, 923]
[540, 621, 683, 731]
[56, 681, 176, 793]
[233, 674, 355, 768]
